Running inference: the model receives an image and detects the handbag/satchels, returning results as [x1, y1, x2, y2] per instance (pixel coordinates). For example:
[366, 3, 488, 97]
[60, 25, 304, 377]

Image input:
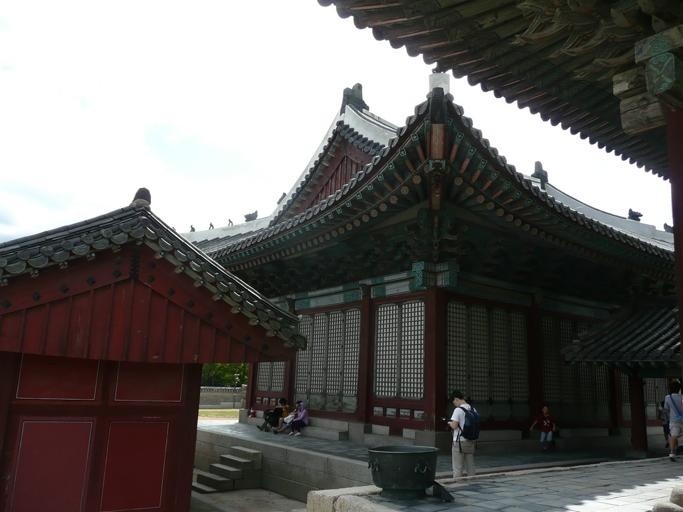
[284, 415, 295, 423]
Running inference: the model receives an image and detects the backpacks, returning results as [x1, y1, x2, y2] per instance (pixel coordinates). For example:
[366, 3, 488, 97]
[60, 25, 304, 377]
[456, 405, 480, 440]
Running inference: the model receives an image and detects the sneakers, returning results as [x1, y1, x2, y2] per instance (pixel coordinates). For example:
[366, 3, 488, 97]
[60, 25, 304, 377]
[670, 453, 676, 461]
[257, 425, 301, 436]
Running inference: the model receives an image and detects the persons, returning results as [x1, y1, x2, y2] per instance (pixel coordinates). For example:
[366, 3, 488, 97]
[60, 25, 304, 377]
[529, 404, 556, 452]
[658, 400, 671, 448]
[444, 389, 479, 478]
[289, 401, 309, 436]
[272, 409, 295, 434]
[662, 380, 683, 461]
[257, 397, 290, 431]
